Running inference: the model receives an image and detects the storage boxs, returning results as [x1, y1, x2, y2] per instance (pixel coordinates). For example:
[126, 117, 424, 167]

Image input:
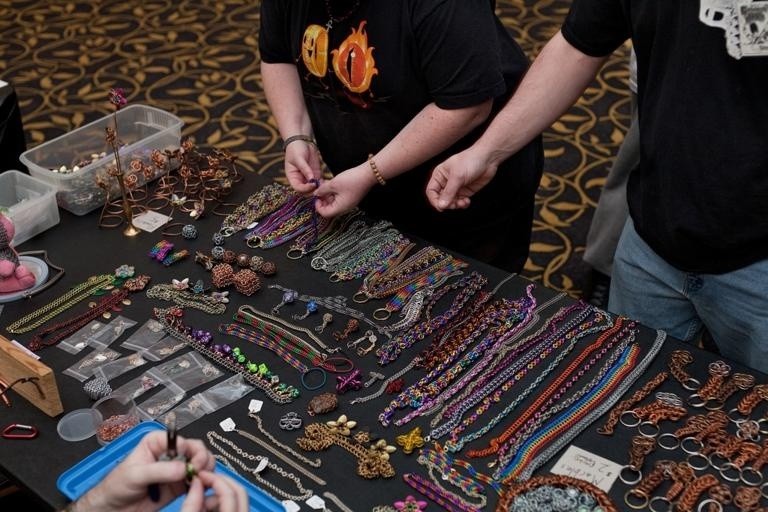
[15, 103, 185, 218]
[0, 170, 62, 245]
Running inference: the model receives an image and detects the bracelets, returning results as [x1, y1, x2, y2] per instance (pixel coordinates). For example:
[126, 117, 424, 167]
[368, 153, 386, 185]
[282, 134, 317, 152]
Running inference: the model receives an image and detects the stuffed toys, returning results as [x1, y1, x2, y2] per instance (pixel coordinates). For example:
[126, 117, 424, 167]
[0, 214, 35, 293]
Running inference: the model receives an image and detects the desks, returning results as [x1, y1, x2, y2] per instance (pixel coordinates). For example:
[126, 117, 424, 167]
[1, 144, 768, 511]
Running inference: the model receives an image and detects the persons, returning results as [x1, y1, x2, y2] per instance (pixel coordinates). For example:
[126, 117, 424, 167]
[60, 429, 249, 512]
[425, 0, 768, 375]
[259, 0, 543, 275]
